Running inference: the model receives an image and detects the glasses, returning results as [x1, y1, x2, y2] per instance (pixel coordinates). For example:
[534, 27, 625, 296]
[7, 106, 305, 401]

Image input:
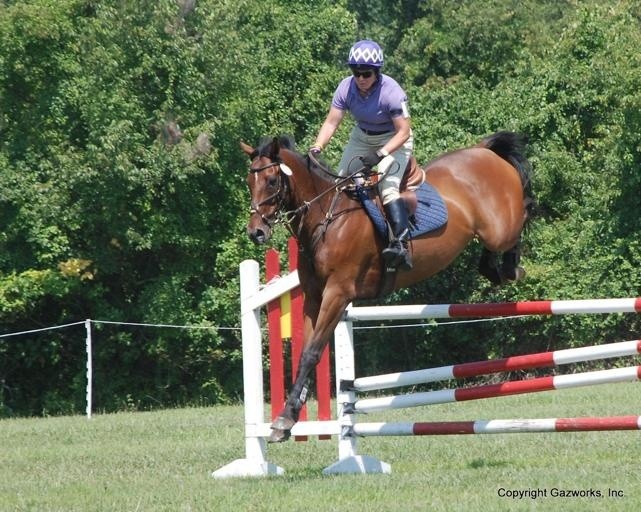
[352, 70, 371, 78]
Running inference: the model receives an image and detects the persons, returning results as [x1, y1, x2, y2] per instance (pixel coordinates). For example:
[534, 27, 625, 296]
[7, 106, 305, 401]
[311, 39, 414, 272]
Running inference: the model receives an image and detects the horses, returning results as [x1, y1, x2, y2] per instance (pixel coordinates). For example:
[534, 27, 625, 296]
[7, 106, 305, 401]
[239, 131, 538, 443]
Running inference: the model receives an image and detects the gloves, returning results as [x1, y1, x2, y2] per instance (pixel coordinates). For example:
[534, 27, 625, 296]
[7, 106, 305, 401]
[359, 151, 384, 168]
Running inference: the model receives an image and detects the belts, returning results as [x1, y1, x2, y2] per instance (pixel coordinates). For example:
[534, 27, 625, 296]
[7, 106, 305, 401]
[361, 128, 389, 135]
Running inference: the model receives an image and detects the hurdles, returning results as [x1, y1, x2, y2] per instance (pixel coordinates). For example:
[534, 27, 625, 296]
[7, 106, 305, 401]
[333, 298, 640, 461]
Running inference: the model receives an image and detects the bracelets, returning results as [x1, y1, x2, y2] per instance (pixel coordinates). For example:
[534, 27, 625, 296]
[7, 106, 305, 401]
[314, 144, 323, 150]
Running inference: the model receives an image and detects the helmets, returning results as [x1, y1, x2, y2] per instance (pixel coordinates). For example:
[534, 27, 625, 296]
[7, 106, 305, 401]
[345, 41, 383, 66]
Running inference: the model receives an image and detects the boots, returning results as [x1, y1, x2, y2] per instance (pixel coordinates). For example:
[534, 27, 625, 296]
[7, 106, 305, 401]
[382, 198, 411, 270]
